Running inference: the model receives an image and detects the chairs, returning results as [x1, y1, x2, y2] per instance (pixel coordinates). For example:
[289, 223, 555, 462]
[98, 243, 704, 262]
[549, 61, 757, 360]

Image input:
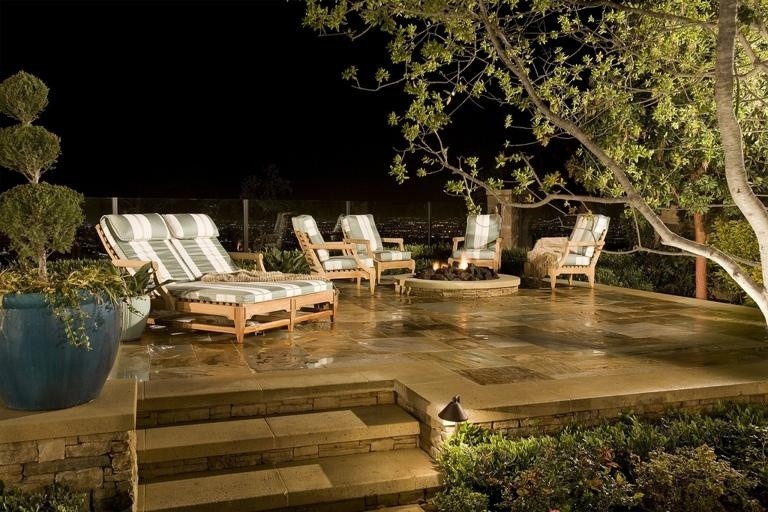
[95, 211, 334, 344]
[525, 213, 610, 288]
[291, 215, 377, 294]
[340, 213, 415, 284]
[447, 213, 503, 273]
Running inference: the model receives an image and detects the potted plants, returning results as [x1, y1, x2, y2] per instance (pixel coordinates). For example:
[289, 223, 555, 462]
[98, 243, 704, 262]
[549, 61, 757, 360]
[0, 72, 142, 410]
[100, 258, 176, 342]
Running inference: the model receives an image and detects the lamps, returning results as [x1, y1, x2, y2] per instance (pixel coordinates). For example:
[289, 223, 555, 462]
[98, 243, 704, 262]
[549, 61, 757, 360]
[437, 392, 467, 423]
